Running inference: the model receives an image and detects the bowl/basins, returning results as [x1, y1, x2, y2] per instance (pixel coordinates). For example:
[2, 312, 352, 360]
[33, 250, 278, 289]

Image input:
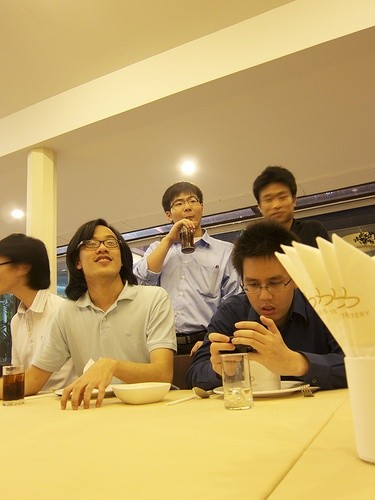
[113, 382, 171, 405]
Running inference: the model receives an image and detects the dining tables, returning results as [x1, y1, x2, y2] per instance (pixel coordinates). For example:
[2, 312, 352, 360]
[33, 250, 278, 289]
[0, 388, 375, 500]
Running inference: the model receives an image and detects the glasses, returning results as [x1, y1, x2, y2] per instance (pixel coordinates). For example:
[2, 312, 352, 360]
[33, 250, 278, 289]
[169, 196, 200, 210]
[240, 278, 292, 293]
[76, 239, 122, 251]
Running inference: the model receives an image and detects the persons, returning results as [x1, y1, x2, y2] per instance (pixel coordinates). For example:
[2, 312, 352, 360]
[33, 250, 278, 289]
[186, 218, 347, 390]
[131, 181, 244, 357]
[0, 232, 78, 394]
[252, 166, 334, 248]
[0, 218, 174, 410]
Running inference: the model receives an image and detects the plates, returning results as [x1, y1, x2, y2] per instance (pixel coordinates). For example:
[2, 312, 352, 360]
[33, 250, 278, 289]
[55, 384, 115, 397]
[214, 381, 310, 398]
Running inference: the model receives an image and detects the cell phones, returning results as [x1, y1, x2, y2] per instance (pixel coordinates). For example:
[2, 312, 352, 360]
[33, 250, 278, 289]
[219, 336, 256, 354]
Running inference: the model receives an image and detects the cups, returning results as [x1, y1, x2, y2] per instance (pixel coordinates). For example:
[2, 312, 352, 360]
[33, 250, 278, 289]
[221, 353, 253, 409]
[345, 357, 375, 464]
[2, 366, 25, 407]
[249, 360, 281, 391]
[179, 225, 196, 254]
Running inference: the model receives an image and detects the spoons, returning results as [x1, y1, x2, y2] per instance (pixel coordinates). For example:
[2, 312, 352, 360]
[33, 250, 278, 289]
[193, 386, 216, 398]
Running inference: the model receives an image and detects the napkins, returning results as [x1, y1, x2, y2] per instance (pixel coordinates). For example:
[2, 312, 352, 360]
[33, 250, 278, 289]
[275, 234, 375, 357]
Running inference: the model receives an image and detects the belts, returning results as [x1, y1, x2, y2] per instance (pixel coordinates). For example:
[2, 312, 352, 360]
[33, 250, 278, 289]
[177, 332, 207, 345]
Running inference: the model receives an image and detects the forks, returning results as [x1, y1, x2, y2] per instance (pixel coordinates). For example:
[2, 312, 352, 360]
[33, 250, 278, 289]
[298, 385, 315, 396]
